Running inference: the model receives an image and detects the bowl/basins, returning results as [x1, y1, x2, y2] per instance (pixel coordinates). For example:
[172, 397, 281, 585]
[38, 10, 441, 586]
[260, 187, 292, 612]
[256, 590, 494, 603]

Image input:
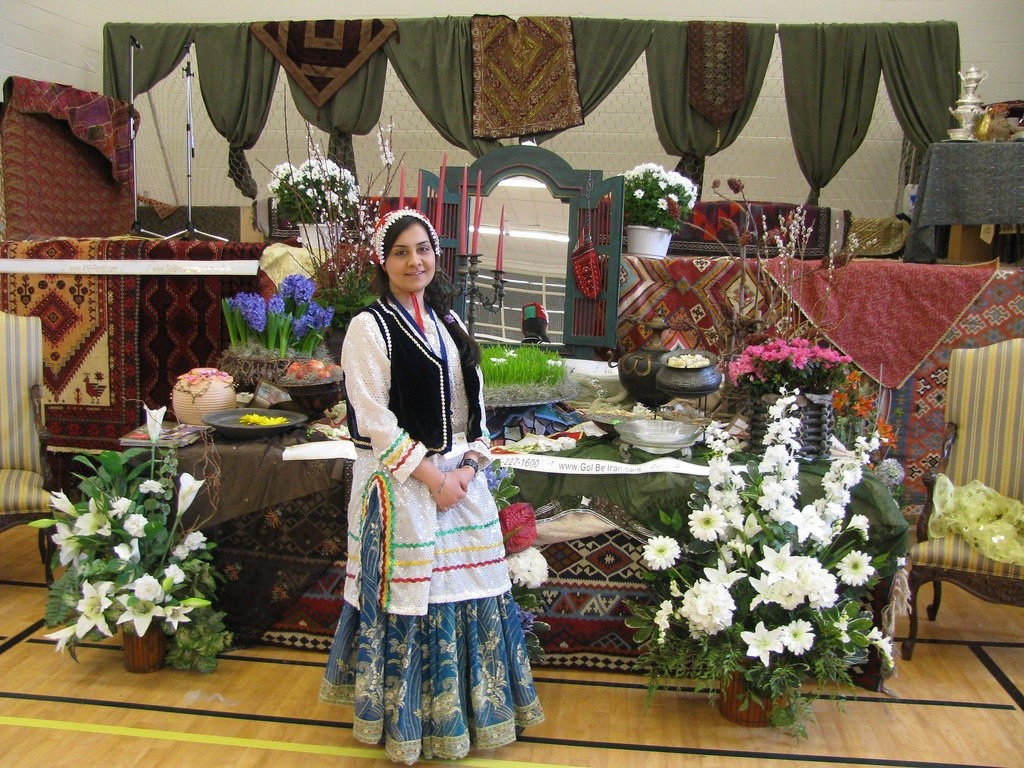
[654, 349, 722, 400]
[613, 419, 705, 455]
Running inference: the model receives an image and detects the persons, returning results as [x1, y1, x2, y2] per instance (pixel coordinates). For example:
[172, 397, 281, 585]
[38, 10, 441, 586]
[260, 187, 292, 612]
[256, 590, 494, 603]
[321, 209, 546, 764]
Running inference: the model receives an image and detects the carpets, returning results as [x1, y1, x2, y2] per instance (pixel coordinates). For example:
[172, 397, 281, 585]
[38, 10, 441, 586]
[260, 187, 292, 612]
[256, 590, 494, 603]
[261, 532, 897, 690]
[1, 236, 271, 451]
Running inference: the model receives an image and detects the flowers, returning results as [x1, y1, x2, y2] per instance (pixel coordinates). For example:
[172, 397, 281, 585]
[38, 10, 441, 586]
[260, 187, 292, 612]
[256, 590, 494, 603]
[26, 406, 235, 673]
[220, 276, 333, 356]
[617, 162, 699, 232]
[269, 154, 359, 227]
[724, 338, 852, 396]
[253, 83, 406, 328]
[623, 382, 907, 740]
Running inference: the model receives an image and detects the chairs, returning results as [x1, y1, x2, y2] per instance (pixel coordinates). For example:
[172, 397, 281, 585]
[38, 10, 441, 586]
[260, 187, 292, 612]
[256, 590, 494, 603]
[898, 338, 1024, 663]
[1, 312, 67, 593]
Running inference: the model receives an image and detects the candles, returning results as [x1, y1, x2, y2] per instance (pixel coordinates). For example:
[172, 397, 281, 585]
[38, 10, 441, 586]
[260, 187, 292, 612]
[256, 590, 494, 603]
[394, 152, 505, 283]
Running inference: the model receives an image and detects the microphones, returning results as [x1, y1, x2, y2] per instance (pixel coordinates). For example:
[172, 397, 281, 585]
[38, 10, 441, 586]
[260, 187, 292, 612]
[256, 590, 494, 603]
[130, 35, 143, 51]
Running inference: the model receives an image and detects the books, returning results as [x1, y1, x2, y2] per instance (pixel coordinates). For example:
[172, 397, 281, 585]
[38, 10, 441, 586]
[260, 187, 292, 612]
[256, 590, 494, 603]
[118, 421, 216, 448]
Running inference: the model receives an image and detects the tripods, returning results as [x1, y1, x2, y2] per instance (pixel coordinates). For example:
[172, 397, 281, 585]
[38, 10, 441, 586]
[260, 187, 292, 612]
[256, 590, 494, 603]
[125, 42, 228, 241]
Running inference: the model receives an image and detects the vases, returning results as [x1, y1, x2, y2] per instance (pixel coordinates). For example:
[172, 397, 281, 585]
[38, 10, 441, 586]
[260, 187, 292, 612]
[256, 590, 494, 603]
[297, 225, 340, 252]
[720, 657, 791, 724]
[752, 393, 834, 464]
[120, 620, 167, 675]
[329, 327, 347, 363]
[625, 224, 670, 259]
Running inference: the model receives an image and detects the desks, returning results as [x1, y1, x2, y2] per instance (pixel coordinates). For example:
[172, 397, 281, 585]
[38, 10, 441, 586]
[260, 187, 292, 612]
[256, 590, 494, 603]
[124, 378, 912, 688]
[902, 141, 1024, 266]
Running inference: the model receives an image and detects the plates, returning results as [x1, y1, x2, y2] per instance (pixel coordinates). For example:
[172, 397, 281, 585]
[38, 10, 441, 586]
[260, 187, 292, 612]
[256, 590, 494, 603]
[201, 406, 309, 440]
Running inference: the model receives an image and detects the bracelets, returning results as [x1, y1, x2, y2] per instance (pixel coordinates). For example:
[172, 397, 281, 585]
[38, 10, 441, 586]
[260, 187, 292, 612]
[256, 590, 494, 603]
[457, 459, 479, 472]
[428, 471, 447, 497]
